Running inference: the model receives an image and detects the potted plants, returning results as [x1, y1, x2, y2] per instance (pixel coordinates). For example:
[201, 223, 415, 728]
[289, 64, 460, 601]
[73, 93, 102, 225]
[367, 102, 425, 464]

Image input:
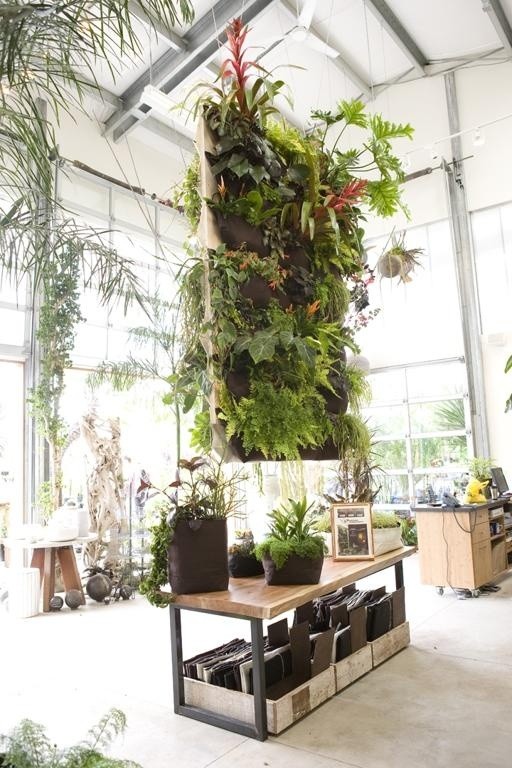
[139, 459, 326, 604]
[314, 511, 405, 559]
[460, 457, 494, 495]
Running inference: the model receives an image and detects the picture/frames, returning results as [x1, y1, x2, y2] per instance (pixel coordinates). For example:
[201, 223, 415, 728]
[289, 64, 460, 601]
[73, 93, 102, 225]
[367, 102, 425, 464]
[330, 501, 375, 563]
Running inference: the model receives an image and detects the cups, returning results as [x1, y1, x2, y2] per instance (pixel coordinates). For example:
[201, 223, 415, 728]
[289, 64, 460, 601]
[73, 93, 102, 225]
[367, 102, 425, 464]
[490, 485, 498, 500]
[490, 522, 504, 534]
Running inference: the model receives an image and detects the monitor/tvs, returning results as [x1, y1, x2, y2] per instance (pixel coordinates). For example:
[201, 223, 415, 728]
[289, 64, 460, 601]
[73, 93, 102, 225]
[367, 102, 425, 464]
[491, 466, 511, 498]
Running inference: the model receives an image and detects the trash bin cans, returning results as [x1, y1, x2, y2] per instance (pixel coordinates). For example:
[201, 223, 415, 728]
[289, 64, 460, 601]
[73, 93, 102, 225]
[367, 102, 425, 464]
[8, 568, 41, 619]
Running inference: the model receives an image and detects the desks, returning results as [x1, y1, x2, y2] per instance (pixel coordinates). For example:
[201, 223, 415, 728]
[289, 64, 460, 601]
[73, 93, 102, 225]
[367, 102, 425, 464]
[3, 532, 99, 614]
[151, 545, 418, 743]
[410, 496, 511, 600]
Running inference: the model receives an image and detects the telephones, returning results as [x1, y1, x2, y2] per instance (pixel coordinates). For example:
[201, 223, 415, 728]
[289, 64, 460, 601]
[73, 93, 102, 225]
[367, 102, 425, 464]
[443, 492, 461, 507]
